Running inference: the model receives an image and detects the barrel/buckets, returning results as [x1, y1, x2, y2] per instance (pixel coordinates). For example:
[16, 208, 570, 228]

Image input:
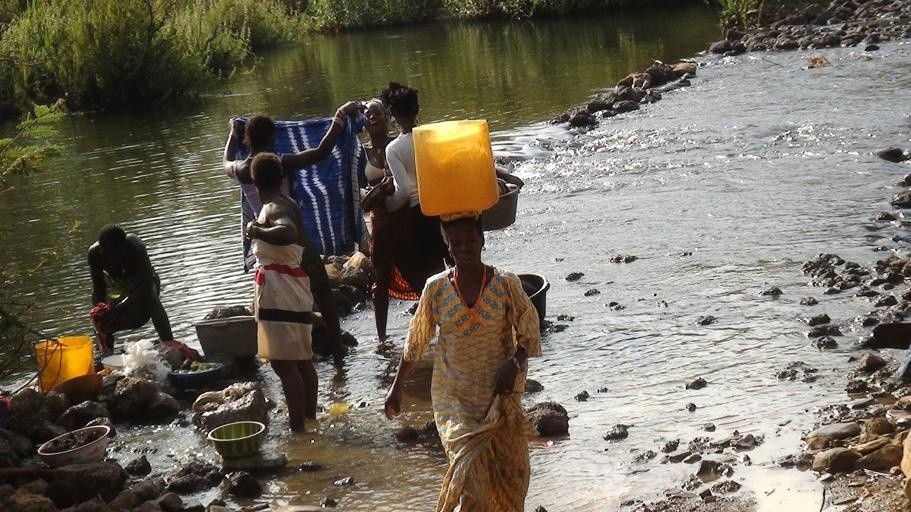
[35, 336, 94, 393]
[412, 118, 498, 216]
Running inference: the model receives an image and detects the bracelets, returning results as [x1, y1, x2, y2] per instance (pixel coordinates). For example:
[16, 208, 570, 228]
[251, 226, 257, 239]
[331, 107, 348, 129]
[511, 355, 522, 372]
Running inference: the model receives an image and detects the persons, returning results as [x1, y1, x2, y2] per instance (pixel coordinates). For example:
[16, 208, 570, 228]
[246, 152, 319, 437]
[370, 82, 456, 290]
[355, 98, 399, 345]
[383, 212, 543, 511]
[220, 99, 359, 366]
[88, 223, 174, 357]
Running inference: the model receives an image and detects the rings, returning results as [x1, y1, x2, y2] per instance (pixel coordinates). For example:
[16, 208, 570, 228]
[505, 389, 510, 392]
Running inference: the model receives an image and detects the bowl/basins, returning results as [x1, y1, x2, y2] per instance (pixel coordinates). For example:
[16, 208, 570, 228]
[482, 185, 520, 229]
[172, 363, 223, 387]
[193, 316, 258, 362]
[38, 425, 111, 468]
[516, 273, 549, 321]
[103, 355, 125, 371]
